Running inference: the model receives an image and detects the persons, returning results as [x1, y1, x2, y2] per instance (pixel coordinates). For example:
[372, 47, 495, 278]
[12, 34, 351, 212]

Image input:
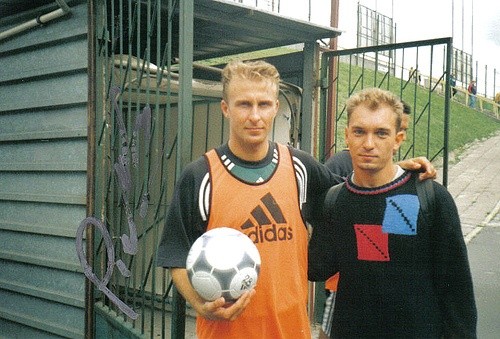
[450, 73, 458, 98]
[156, 59, 437, 339]
[468, 79, 477, 110]
[305, 100, 412, 339]
[409, 66, 421, 85]
[307, 87, 478, 339]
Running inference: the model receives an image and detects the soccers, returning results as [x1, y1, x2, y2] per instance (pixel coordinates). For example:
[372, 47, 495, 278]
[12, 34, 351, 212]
[186, 227, 261, 304]
